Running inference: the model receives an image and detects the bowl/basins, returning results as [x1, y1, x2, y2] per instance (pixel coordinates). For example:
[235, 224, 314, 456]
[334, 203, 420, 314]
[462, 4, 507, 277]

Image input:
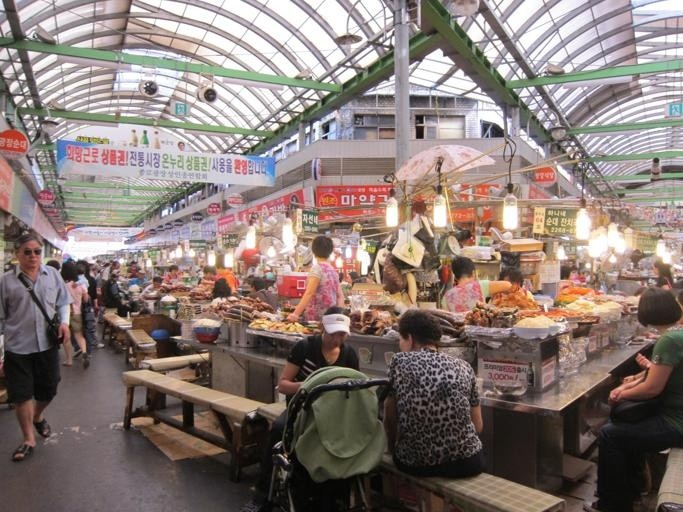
[515, 328, 549, 339]
[197, 334, 218, 343]
[161, 300, 177, 306]
[150, 329, 169, 340]
[493, 382, 526, 398]
[194, 327, 220, 334]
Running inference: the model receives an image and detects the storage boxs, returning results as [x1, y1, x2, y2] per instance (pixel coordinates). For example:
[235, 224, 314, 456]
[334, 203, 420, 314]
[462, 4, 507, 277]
[539, 261, 561, 283]
[473, 261, 501, 278]
[476, 338, 561, 394]
[276, 271, 309, 298]
[501, 254, 544, 274]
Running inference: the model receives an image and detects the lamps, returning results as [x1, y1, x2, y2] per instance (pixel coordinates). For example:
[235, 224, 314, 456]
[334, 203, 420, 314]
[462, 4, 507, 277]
[145, 255, 152, 267]
[334, 0, 388, 46]
[345, 241, 351, 258]
[358, 240, 362, 252]
[189, 245, 195, 258]
[526, 108, 566, 141]
[385, 188, 398, 228]
[246, 219, 256, 249]
[13, 94, 59, 134]
[655, 234, 666, 256]
[282, 212, 293, 244]
[432, 184, 447, 227]
[503, 183, 518, 231]
[574, 199, 590, 240]
[176, 241, 182, 257]
[608, 252, 617, 263]
[608, 215, 617, 247]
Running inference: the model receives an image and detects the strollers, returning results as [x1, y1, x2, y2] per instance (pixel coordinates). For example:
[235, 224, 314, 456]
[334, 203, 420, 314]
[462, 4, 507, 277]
[262, 362, 396, 512]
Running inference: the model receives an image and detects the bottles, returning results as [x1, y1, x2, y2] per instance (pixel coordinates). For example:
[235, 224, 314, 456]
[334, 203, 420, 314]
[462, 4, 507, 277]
[527, 361, 534, 388]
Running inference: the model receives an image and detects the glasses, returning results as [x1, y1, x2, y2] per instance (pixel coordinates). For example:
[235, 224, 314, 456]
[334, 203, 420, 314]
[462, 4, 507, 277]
[19, 248, 42, 255]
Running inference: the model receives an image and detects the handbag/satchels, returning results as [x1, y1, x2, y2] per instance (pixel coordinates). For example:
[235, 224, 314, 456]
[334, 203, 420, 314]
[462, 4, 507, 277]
[46, 312, 64, 350]
[609, 396, 660, 426]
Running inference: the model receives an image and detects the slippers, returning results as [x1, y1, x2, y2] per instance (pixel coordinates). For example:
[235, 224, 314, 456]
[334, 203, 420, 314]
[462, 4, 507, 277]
[9, 443, 35, 462]
[33, 418, 51, 438]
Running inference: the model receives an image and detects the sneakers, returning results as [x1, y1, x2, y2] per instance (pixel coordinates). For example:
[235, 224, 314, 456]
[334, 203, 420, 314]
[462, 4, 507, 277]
[72, 349, 83, 358]
[582, 497, 628, 511]
[593, 486, 643, 505]
[640, 488, 649, 496]
[89, 343, 105, 349]
[81, 352, 90, 369]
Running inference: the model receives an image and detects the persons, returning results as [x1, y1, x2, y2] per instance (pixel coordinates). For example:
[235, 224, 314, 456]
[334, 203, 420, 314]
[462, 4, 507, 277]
[96, 259, 276, 318]
[380, 309, 484, 480]
[239, 306, 360, 511]
[288, 233, 530, 319]
[561, 250, 683, 511]
[2, 234, 106, 464]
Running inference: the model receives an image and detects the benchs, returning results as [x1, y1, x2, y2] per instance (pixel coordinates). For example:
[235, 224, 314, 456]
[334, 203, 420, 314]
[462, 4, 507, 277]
[259, 400, 568, 511]
[103, 307, 118, 338]
[120, 370, 269, 482]
[139, 351, 211, 405]
[653, 448, 682, 511]
[104, 312, 132, 341]
[126, 329, 158, 371]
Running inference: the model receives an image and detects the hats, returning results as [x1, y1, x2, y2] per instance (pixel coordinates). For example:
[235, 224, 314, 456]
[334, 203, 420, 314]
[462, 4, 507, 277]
[321, 313, 351, 336]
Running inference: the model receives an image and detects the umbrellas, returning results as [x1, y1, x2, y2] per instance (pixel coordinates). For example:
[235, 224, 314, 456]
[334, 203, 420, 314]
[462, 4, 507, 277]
[394, 145, 496, 231]
